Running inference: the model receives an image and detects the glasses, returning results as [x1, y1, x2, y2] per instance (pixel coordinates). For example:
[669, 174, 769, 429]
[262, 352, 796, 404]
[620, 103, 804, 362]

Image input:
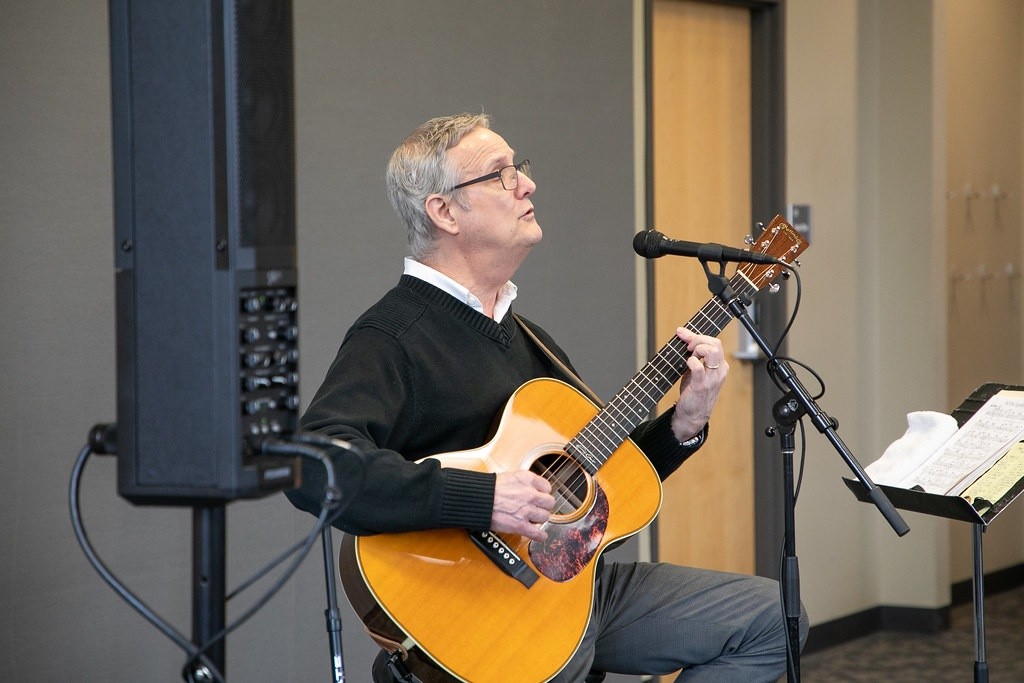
[446, 159, 533, 193]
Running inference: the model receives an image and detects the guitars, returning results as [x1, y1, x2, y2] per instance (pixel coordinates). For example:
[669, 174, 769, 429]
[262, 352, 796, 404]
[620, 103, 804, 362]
[338, 212, 812, 683]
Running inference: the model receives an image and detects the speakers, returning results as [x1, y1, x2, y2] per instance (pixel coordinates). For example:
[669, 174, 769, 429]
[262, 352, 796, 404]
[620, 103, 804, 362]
[109, 0, 302, 504]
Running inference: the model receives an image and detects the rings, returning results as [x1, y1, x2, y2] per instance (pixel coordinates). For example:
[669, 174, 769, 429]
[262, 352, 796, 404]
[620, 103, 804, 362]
[703, 362, 719, 369]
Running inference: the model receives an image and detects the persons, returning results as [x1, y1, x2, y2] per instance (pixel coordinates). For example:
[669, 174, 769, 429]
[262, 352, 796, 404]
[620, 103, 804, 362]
[282, 115, 811, 683]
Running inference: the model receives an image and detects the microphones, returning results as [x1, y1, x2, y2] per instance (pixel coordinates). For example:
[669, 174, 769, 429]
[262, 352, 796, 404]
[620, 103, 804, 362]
[632, 228, 778, 265]
